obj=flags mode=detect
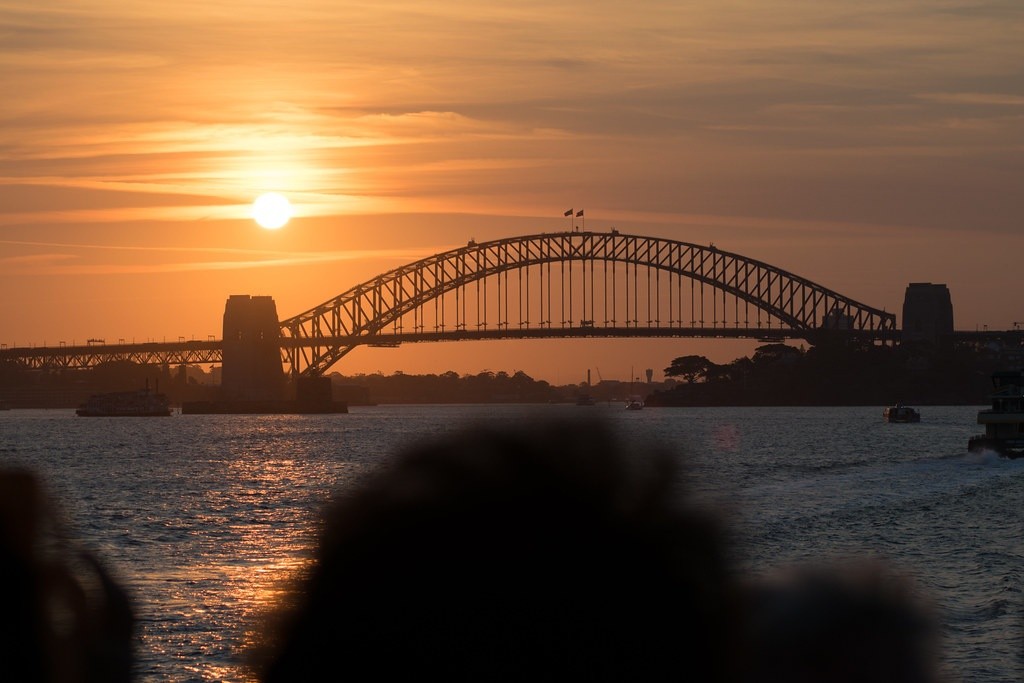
[576,210,583,217]
[564,208,573,216]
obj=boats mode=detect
[576,398,595,406]
[75,375,173,416]
[883,399,921,424]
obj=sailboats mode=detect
[625,366,642,410]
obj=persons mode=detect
[0,403,938,683]
